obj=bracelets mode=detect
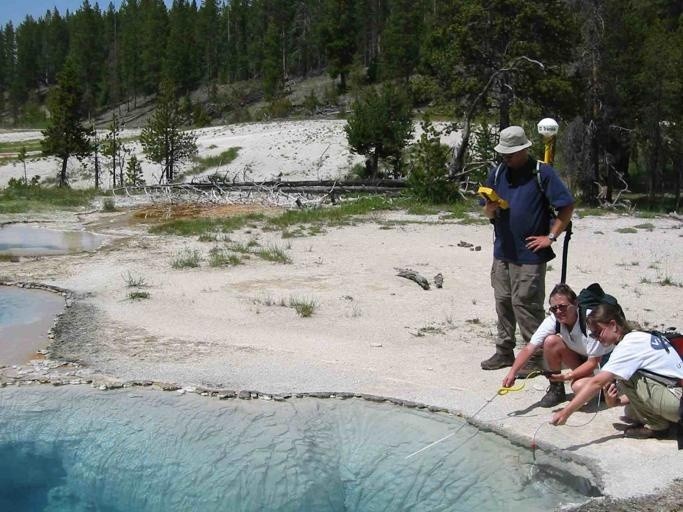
[567,372,572,380]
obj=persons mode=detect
[546,302,681,439]
[501,284,616,408]
[479,126,575,370]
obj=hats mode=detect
[493,125,532,154]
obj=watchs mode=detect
[548,232,555,241]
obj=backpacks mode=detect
[554,282,626,338]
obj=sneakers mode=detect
[513,348,544,379]
[480,349,515,370]
[624,424,670,440]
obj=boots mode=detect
[540,370,566,408]
